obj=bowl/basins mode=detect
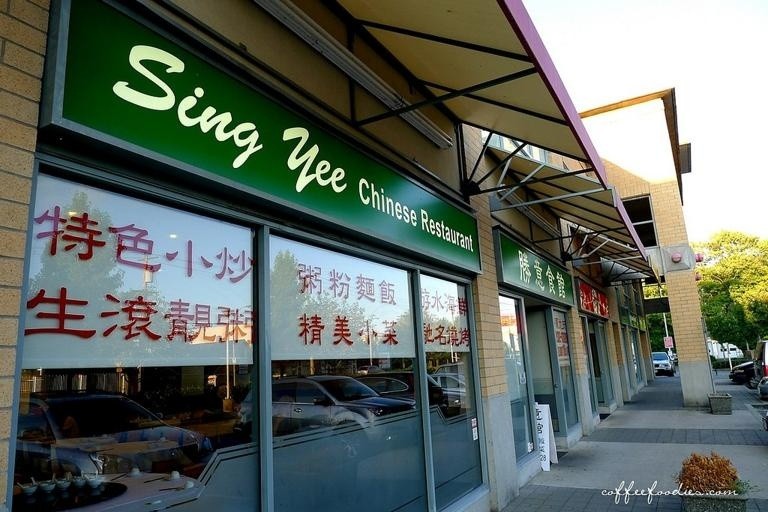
[21,476,102,496]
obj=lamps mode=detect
[497,183,566,241]
[258,0,455,151]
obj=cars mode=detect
[729,360,759,389]
[761,411,768,430]
[431,373,469,405]
[758,376,768,400]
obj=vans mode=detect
[750,340,767,388]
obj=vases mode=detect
[706,392,733,415]
[678,491,748,512]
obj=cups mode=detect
[171,470,180,478]
[160,437,165,441]
[130,467,140,475]
[184,481,193,490]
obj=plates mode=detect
[127,473,144,477]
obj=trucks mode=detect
[706,338,743,360]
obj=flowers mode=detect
[671,450,762,493]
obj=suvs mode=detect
[503,341,523,382]
[231,376,419,437]
[633,356,637,375]
[434,361,468,379]
[15,392,213,475]
[355,372,448,413]
[651,352,675,375]
